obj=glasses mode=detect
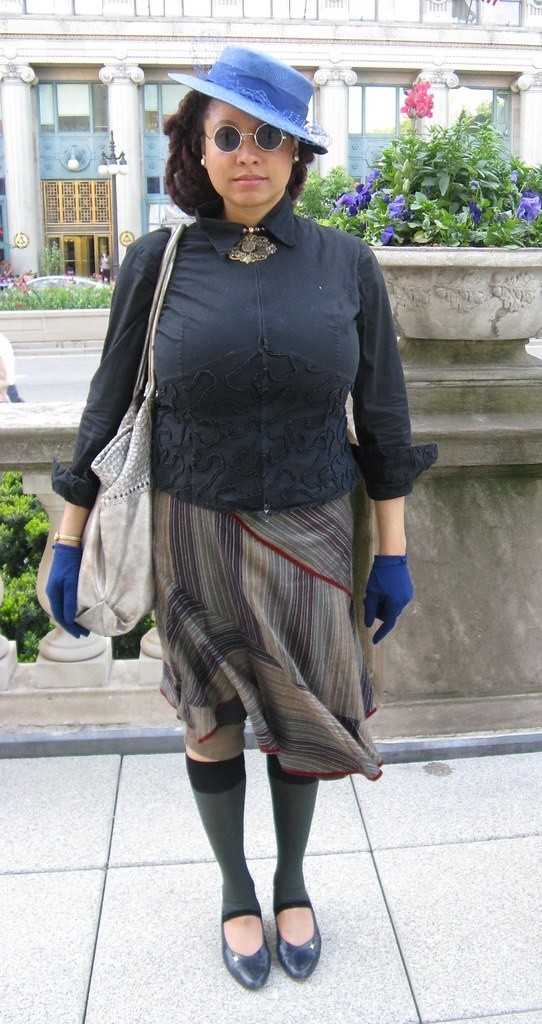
[204,122,288,153]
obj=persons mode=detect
[46,44,441,991]
[2,261,14,280]
[100,250,110,283]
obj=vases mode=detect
[368,249,542,342]
[0,309,113,347]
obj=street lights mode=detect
[97,130,128,281]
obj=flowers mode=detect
[0,278,111,311]
[309,80,542,249]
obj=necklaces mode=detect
[243,226,266,234]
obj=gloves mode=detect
[46,543,89,639]
[365,553,413,644]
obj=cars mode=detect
[23,277,110,294]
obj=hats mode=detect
[166,45,332,156]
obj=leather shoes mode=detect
[220,909,270,989]
[275,899,321,982]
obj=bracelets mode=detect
[53,531,82,541]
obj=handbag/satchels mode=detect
[74,383,158,637]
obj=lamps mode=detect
[66,145,78,171]
